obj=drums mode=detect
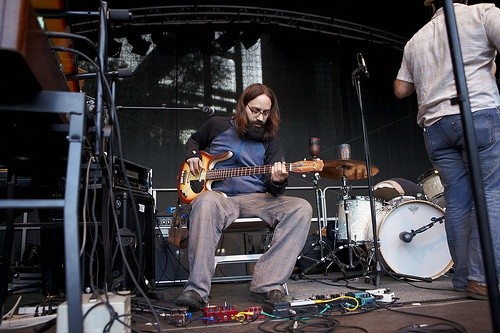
[417,169,447,209]
[369,197,455,281]
[335,195,385,244]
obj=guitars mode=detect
[176,150,324,204]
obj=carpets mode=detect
[282,270,468,306]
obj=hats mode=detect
[424,0,433,6]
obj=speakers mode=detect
[155,217,192,284]
[75,185,156,299]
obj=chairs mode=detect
[215,214,290,296]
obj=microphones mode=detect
[360,54,369,78]
[203,107,215,116]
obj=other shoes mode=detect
[174,290,206,310]
[465,281,488,300]
[250,289,293,306]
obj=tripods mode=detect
[293,172,376,280]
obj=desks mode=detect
[0,86,86,333]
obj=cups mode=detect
[338,144,351,160]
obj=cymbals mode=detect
[320,159,379,180]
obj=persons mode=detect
[395,0,500,301]
[173,83,313,310]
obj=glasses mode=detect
[248,104,272,117]
